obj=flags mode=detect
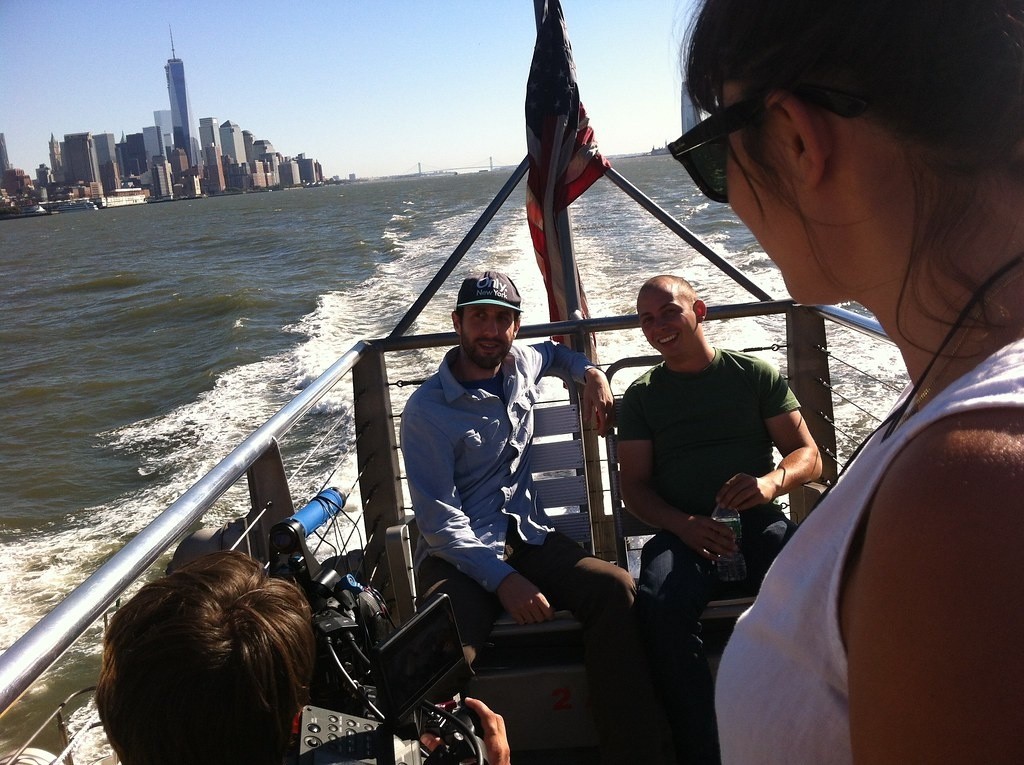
[522,0,609,354]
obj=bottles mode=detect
[712,501,748,584]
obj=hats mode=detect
[456,271,524,313]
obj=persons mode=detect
[399,271,666,763]
[96,552,511,765]
[671,0,1024,764]
[615,276,823,764]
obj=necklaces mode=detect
[887,246,1022,435]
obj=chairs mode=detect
[382,366,624,680]
[598,355,834,625]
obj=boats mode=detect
[50,198,98,213]
[99,186,148,208]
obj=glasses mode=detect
[667,84,871,204]
